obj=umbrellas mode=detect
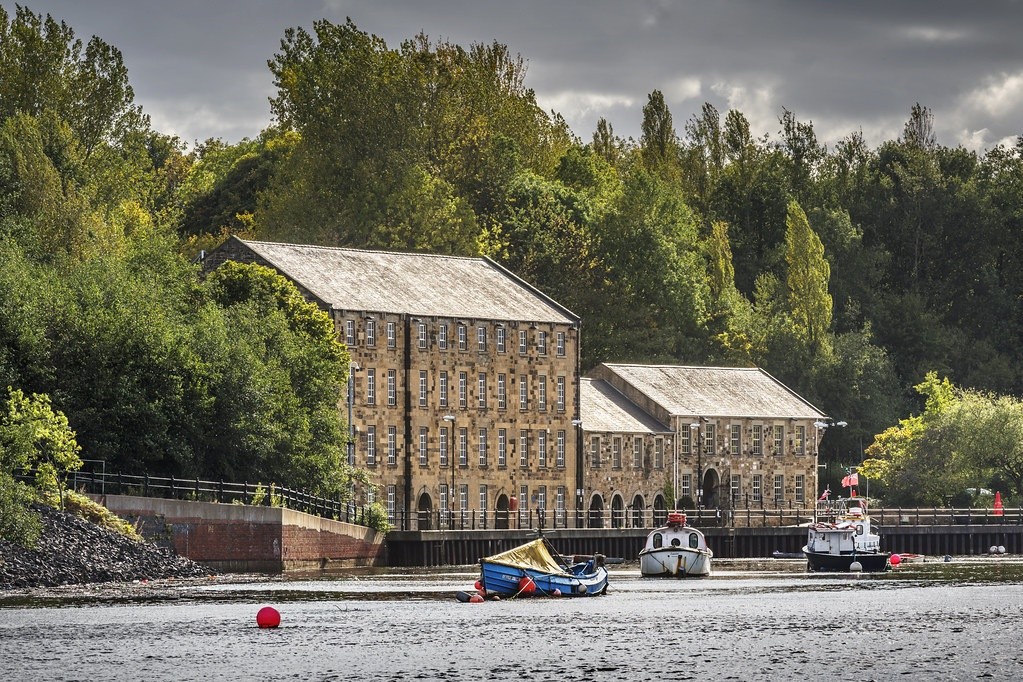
[994,491,1001,516]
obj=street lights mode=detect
[571,420,584,528]
[690,416,708,527]
[442,415,455,530]
[812,421,848,499]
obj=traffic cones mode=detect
[994,490,1003,514]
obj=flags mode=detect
[841,473,857,488]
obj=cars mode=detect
[950,488,993,508]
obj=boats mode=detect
[478,534,609,598]
[802,467,891,573]
[896,554,925,562]
[638,512,713,577]
